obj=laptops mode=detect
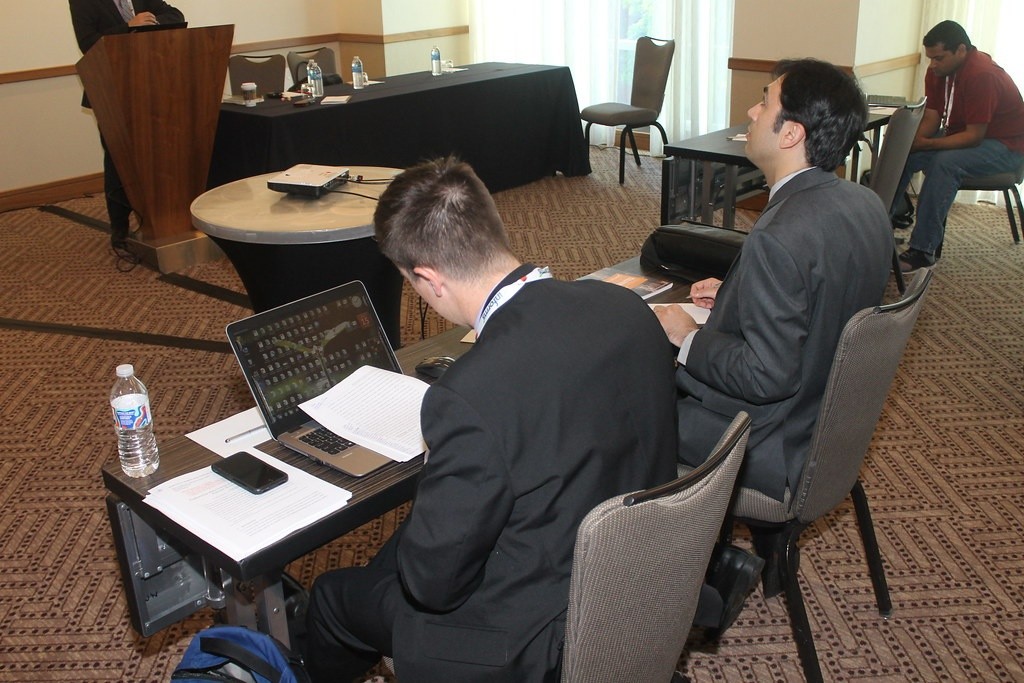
[224,279,402,475]
[128,22,189,33]
[851,65,907,106]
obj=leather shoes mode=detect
[751,520,800,597]
[707,542,764,640]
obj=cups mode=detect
[302,84,316,102]
[241,83,257,107]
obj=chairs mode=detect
[672,265,935,683]
[286,44,337,86]
[580,36,674,183]
[225,54,284,97]
[558,410,754,683]
[864,93,930,295]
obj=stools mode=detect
[933,171,1024,260]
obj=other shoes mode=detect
[111,220,129,248]
[890,247,938,274]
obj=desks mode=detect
[206,61,591,197]
[660,108,890,232]
[102,253,721,652]
[189,166,408,352]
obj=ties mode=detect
[119,0,134,24]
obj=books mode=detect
[732,133,747,142]
[223,95,264,106]
[647,303,711,324]
[281,92,309,98]
[320,96,351,105]
[570,267,674,301]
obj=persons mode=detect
[68,0,186,251]
[675,56,896,646]
[278,154,682,682]
[888,19,1024,274]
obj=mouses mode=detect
[415,355,455,378]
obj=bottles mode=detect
[352,56,364,89]
[109,364,160,479]
[431,45,441,76]
[306,59,323,97]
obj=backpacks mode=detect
[168,626,309,683]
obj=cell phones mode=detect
[212,451,288,494]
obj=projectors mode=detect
[267,164,349,198]
